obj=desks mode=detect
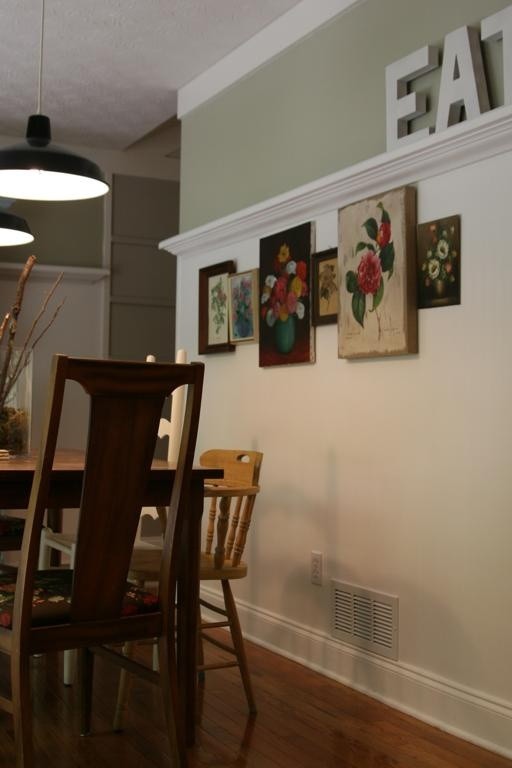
[1,437,226,766]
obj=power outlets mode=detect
[310,552,324,586]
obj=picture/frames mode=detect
[311,247,338,325]
[226,268,259,345]
[199,260,236,354]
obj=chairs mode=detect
[1,353,209,762]
[111,443,262,736]
[31,413,172,691]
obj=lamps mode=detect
[0,0,111,204]
[0,211,36,247]
